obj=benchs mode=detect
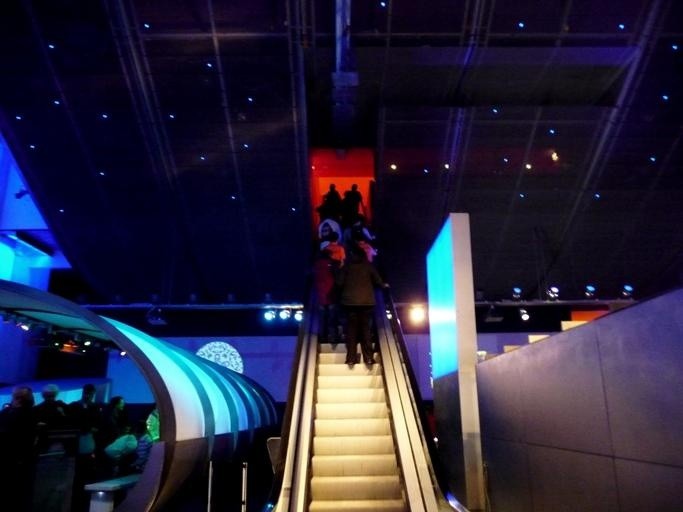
[84,474,144,512]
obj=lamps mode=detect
[511,284,634,300]
[3,313,34,333]
[147,308,168,327]
[518,306,531,322]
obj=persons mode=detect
[314,182,379,344]
[32,383,75,511]
[0,385,37,510]
[67,382,103,511]
[423,403,439,448]
[93,394,159,482]
[335,248,391,366]
